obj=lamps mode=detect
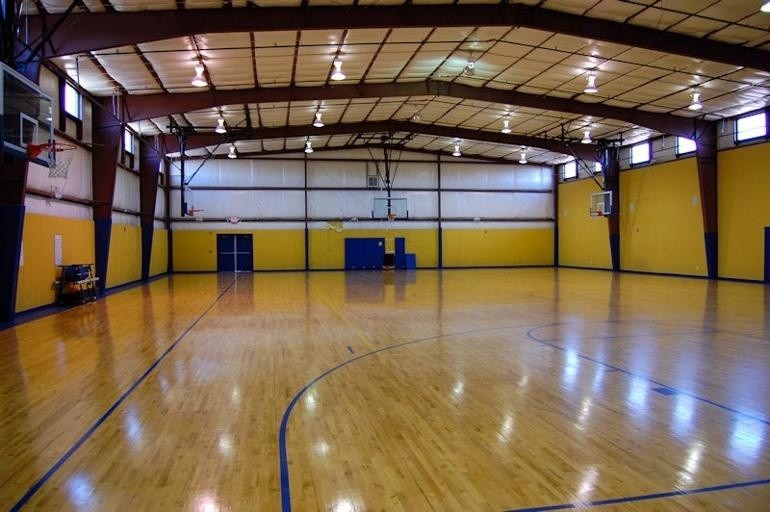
[451,115,529,164]
[579,125,592,144]
[688,87,704,110]
[412,109,420,123]
[190,63,237,159]
[583,72,597,94]
[464,61,475,77]
[305,58,347,154]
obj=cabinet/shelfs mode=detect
[53,263,99,304]
